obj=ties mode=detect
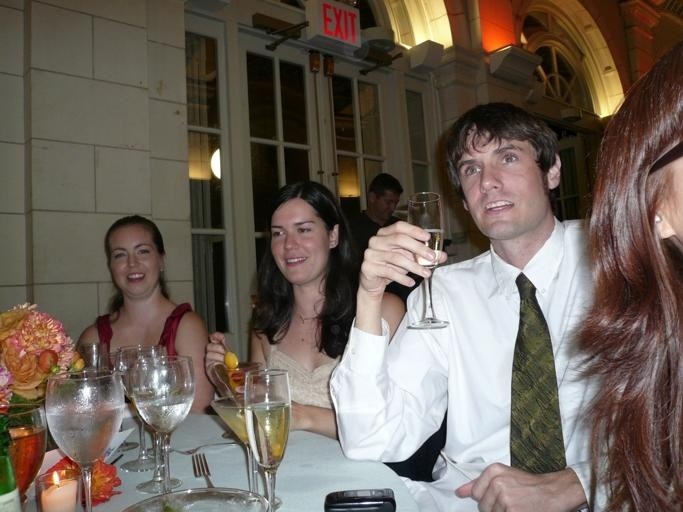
[508,271,568,476]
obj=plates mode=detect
[122,486,269,512]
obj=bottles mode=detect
[0,414,23,512]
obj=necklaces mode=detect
[298,316,315,324]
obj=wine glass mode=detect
[44,369,126,512]
[212,357,291,512]
[73,342,196,495]
[406,191,450,329]
[1,403,47,501]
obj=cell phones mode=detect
[324,488,397,512]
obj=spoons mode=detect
[219,339,239,369]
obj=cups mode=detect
[35,470,84,512]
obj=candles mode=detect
[40,468,78,511]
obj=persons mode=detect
[76,214,214,415]
[569,36,682,510]
[201,178,411,478]
[329,99,618,511]
[348,173,404,254]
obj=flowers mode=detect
[0,303,86,426]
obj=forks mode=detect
[191,452,214,488]
[174,442,241,455]
